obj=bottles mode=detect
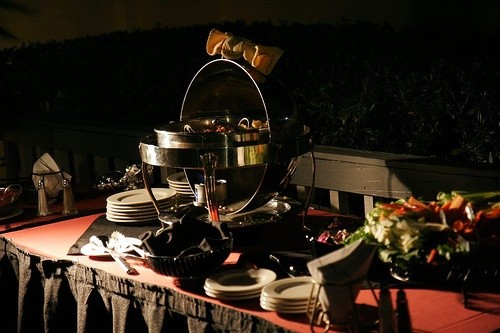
[63,178,77,214]
[396,290,412,333]
[37,180,52,216]
[379,288,395,333]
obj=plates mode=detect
[81,235,142,257]
[167,173,196,203]
[204,268,276,301]
[0,208,24,220]
[105,188,177,223]
[260,277,321,311]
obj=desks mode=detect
[0,211,500,333]
